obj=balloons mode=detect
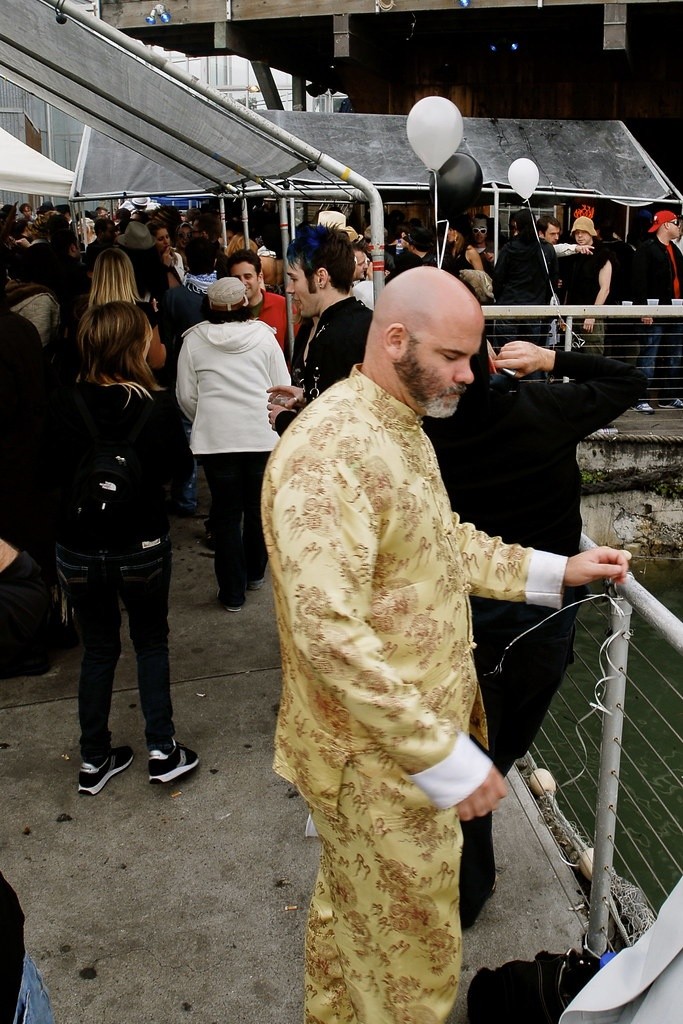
[507,158,539,199]
[406,96,464,173]
[429,152,483,221]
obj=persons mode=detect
[266,222,374,438]
[48,299,201,795]
[175,277,291,612]
[0,536,61,1024]
[262,267,632,1024]
[0,195,683,557]
[422,337,650,934]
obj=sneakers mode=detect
[148,738,199,786]
[83,745,135,799]
[631,402,655,415]
[657,396,683,408]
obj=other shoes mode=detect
[217,588,240,611]
[246,577,265,589]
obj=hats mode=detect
[116,221,156,249]
[403,227,435,249]
[308,211,358,243]
[132,196,150,207]
[570,216,598,237]
[648,211,677,234]
[207,277,248,311]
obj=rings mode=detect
[501,366,517,378]
[579,248,582,249]
[293,397,299,404]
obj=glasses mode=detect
[178,232,192,239]
[472,227,488,234]
[667,221,679,227]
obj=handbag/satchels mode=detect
[467,945,601,1024]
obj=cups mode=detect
[647,299,659,305]
[622,301,633,305]
[272,397,292,430]
[671,298,683,305]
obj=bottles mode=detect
[396,239,403,254]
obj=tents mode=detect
[0,0,683,362]
